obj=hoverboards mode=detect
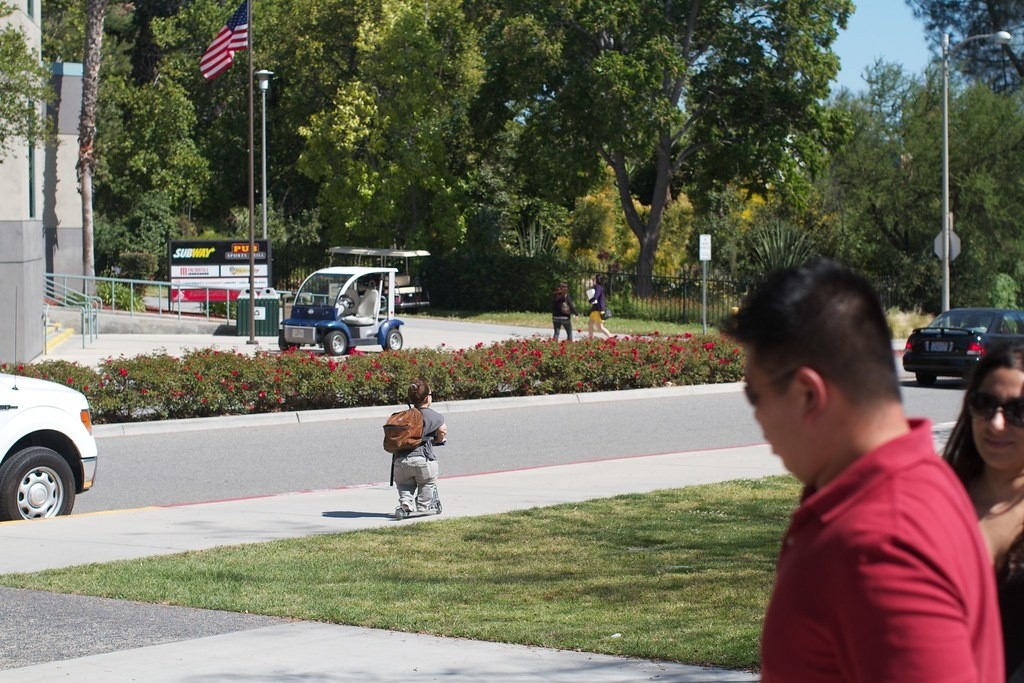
[393,439,446,521]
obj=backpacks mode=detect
[382,408,431,453]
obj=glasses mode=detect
[744,372,794,406]
[967,392,1024,428]
[428,394,434,396]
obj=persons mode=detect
[552,283,578,341]
[588,275,613,338]
[941,350,1024,683]
[394,382,447,512]
[719,266,1005,683]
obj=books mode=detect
[587,289,597,304]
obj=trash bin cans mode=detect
[236,287,280,337]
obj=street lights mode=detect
[254,68,274,241]
[941,29,1013,325]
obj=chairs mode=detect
[343,280,379,325]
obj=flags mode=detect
[200,0,249,80]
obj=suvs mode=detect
[0,372,100,523]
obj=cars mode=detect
[902,306,1024,389]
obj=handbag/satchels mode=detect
[562,293,572,315]
[602,307,612,320]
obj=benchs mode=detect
[384,275,423,294]
